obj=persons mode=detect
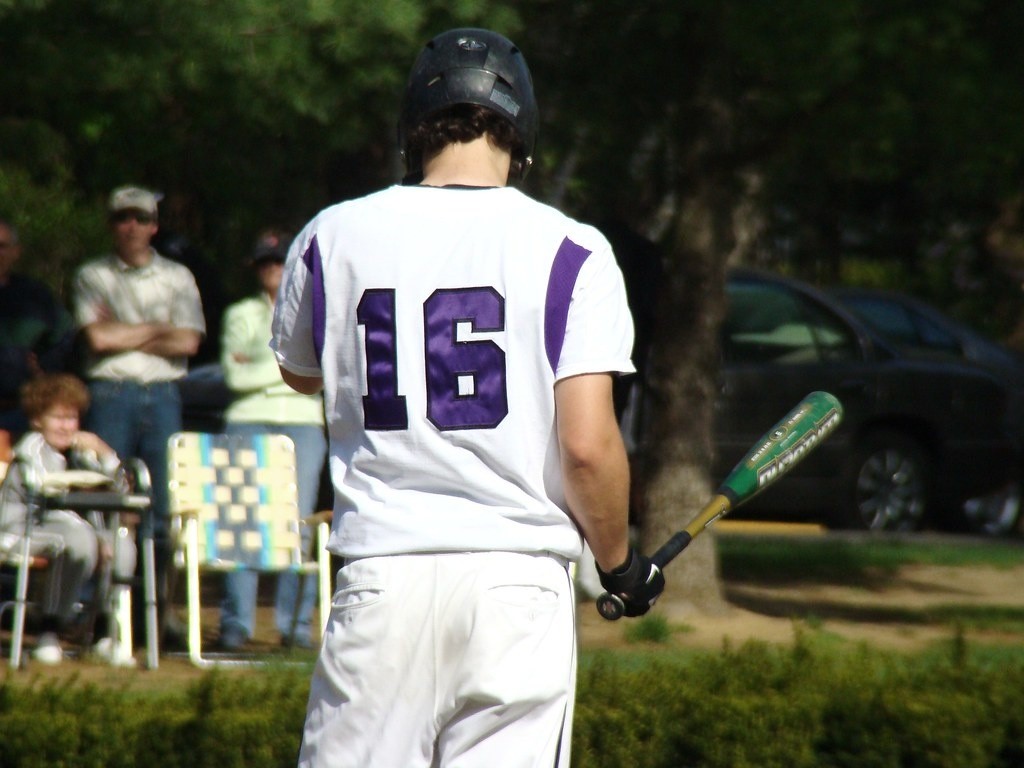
[270,26,664,768]
[218,230,328,653]
[70,186,206,648]
[0,372,141,667]
[0,222,75,477]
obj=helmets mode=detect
[398,28,538,181]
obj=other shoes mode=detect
[30,626,64,666]
[219,624,250,650]
[88,639,137,668]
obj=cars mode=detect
[724,262,1024,537]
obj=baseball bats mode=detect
[596,391,843,622]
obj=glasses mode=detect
[258,255,285,265]
[111,210,154,223]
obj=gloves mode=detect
[596,544,665,617]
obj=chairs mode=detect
[0,430,333,672]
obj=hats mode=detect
[108,184,162,210]
[252,230,293,257]
